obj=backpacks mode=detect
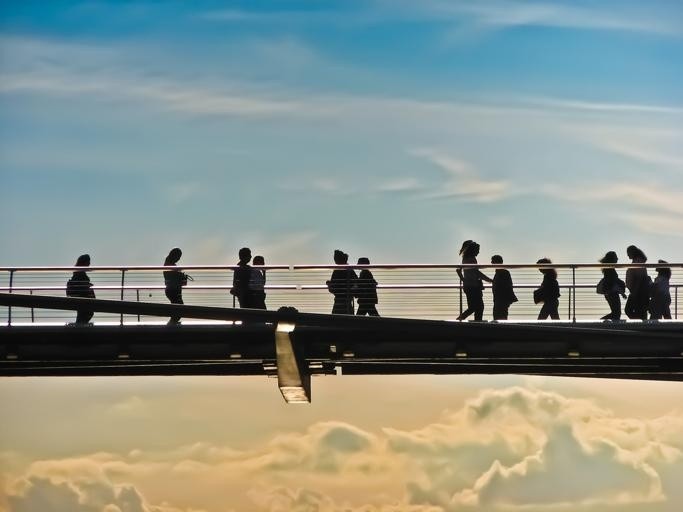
[455,266,466,282]
[348,270,358,298]
[66,275,80,297]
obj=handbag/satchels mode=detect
[88,284,96,298]
[648,276,654,290]
[178,272,193,286]
[534,288,544,304]
[625,294,646,319]
[597,278,625,294]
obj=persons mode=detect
[325,249,358,315]
[648,260,671,319]
[248,255,267,325]
[626,245,648,320]
[350,257,379,316]
[490,255,518,320]
[162,247,183,321]
[229,247,252,326]
[599,251,626,320]
[65,254,96,322]
[533,259,560,320]
[456,240,493,321]
